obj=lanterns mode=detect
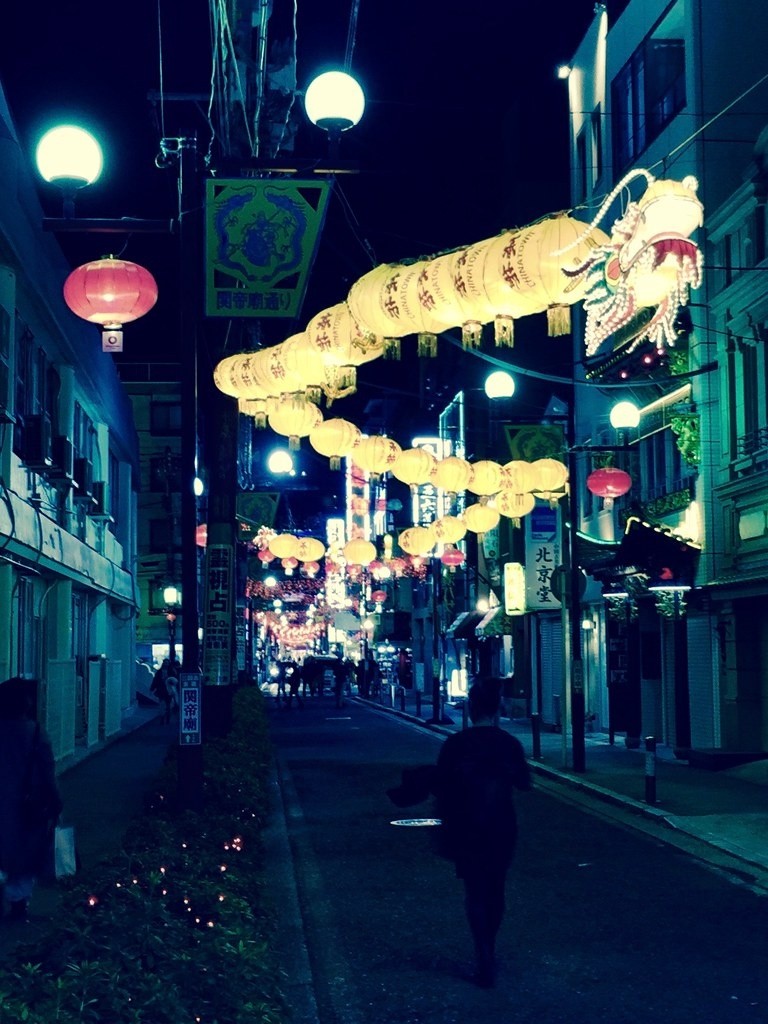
[214,210,632,613]
[196,523,207,554]
[63,254,158,352]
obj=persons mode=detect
[149,656,386,728]
[426,679,533,989]
[0,677,66,923]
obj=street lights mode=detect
[484,371,640,770]
[31,69,368,780]
